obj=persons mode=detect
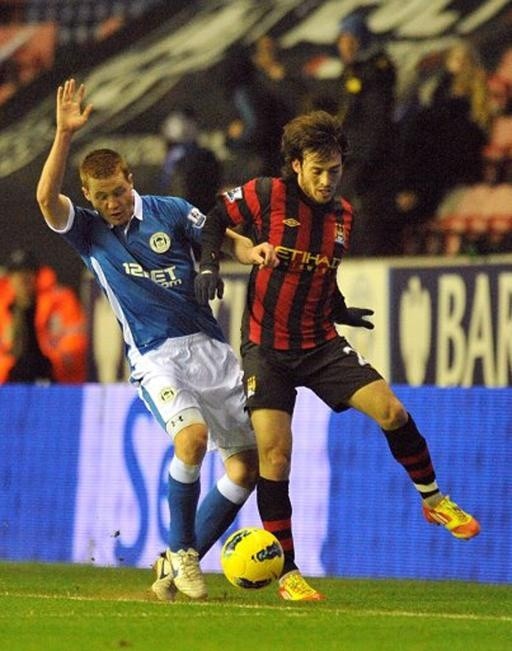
[0,245,96,389]
[195,108,481,603]
[151,16,512,258]
[34,76,260,601]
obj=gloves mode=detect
[194,265,224,304]
[335,303,374,329]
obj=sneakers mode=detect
[152,557,177,602]
[278,569,327,602]
[423,494,480,539]
[167,547,208,598]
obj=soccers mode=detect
[224,528,285,590]
